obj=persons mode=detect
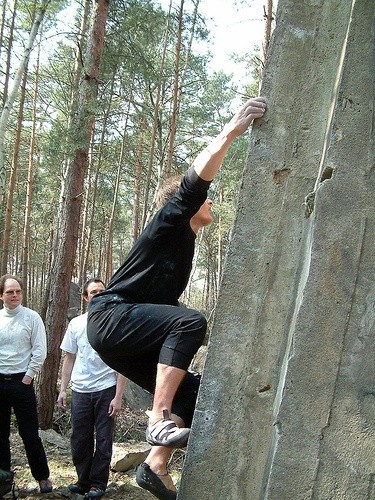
[86,96,267,500]
[57,278,127,500]
[0,274,53,497]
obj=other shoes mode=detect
[136,463,177,500]
[86,487,105,496]
[146,410,190,448]
[39,480,53,493]
[68,484,89,497]
